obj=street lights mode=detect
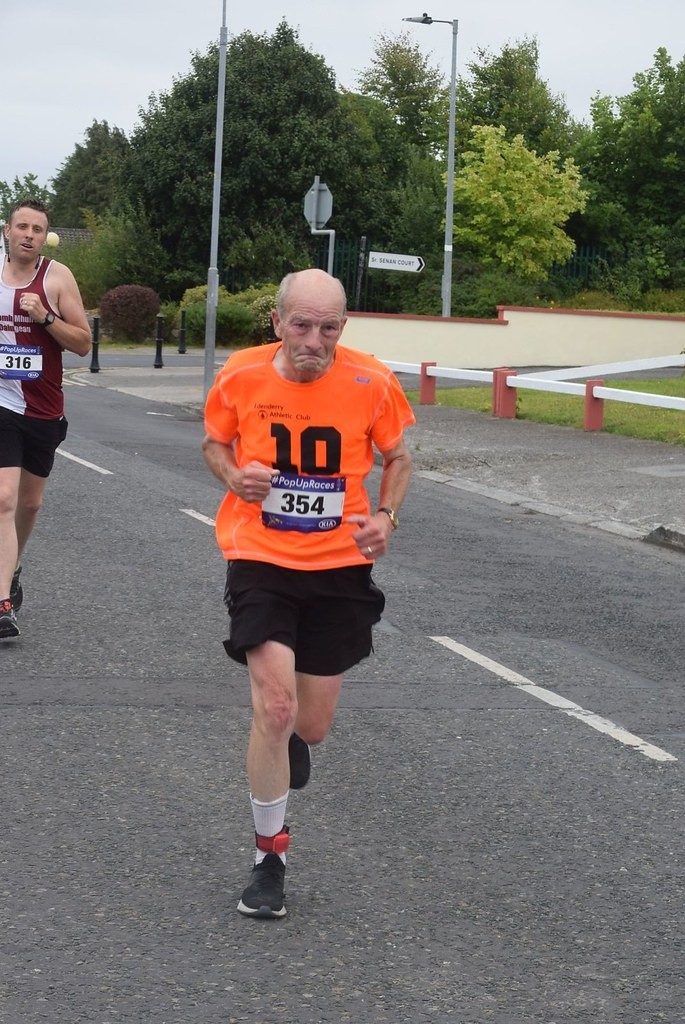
[46,232,59,259]
[404,16,458,318]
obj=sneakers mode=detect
[235,825,290,917]
[0,565,23,638]
[288,732,311,790]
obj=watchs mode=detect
[41,312,55,327]
[378,508,400,529]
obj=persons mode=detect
[0,201,92,639]
[203,269,416,919]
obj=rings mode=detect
[367,546,373,553]
